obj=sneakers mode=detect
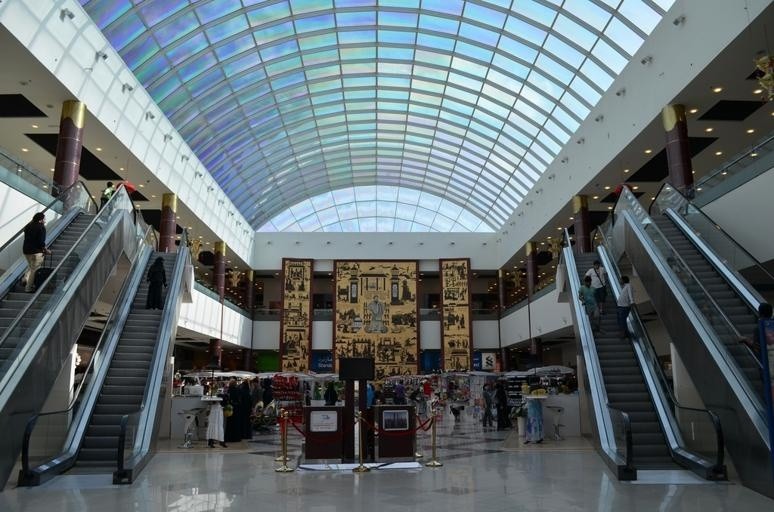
[19,281,27,287]
[25,288,35,292]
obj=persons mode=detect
[96,182,121,217]
[523,400,543,444]
[225,376,464,442]
[146,256,167,310]
[206,402,228,448]
[22,212,50,292]
[578,277,600,338]
[616,275,633,338]
[494,383,513,432]
[483,383,493,427]
[584,260,608,312]
[737,303,774,401]
[368,295,385,331]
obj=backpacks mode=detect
[101,187,113,200]
[150,265,163,282]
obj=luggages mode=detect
[34,253,56,294]
